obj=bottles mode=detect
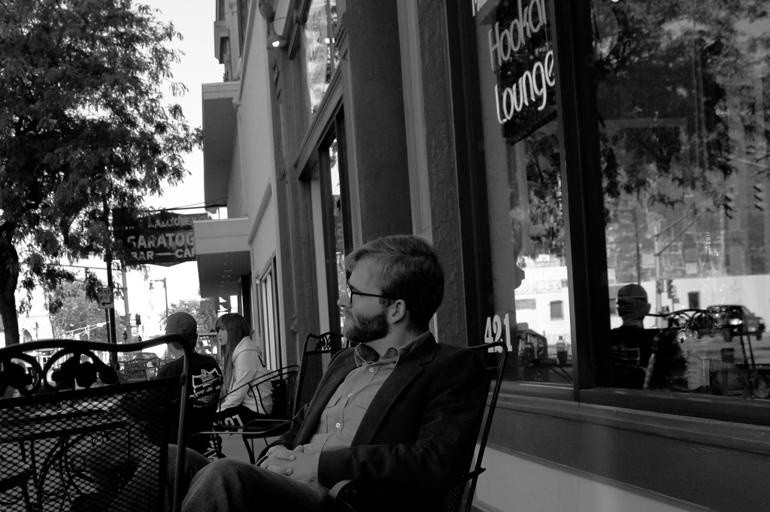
[556,336,568,364]
[711,346,751,397]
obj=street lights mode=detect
[138,336,142,353]
[122,331,128,361]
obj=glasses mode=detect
[344,279,398,307]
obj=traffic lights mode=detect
[656,279,664,294]
[666,279,676,297]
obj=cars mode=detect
[692,305,765,342]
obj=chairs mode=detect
[0,329,509,510]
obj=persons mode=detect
[117,312,223,452]
[610,284,690,393]
[107,234,492,512]
[209,313,274,428]
